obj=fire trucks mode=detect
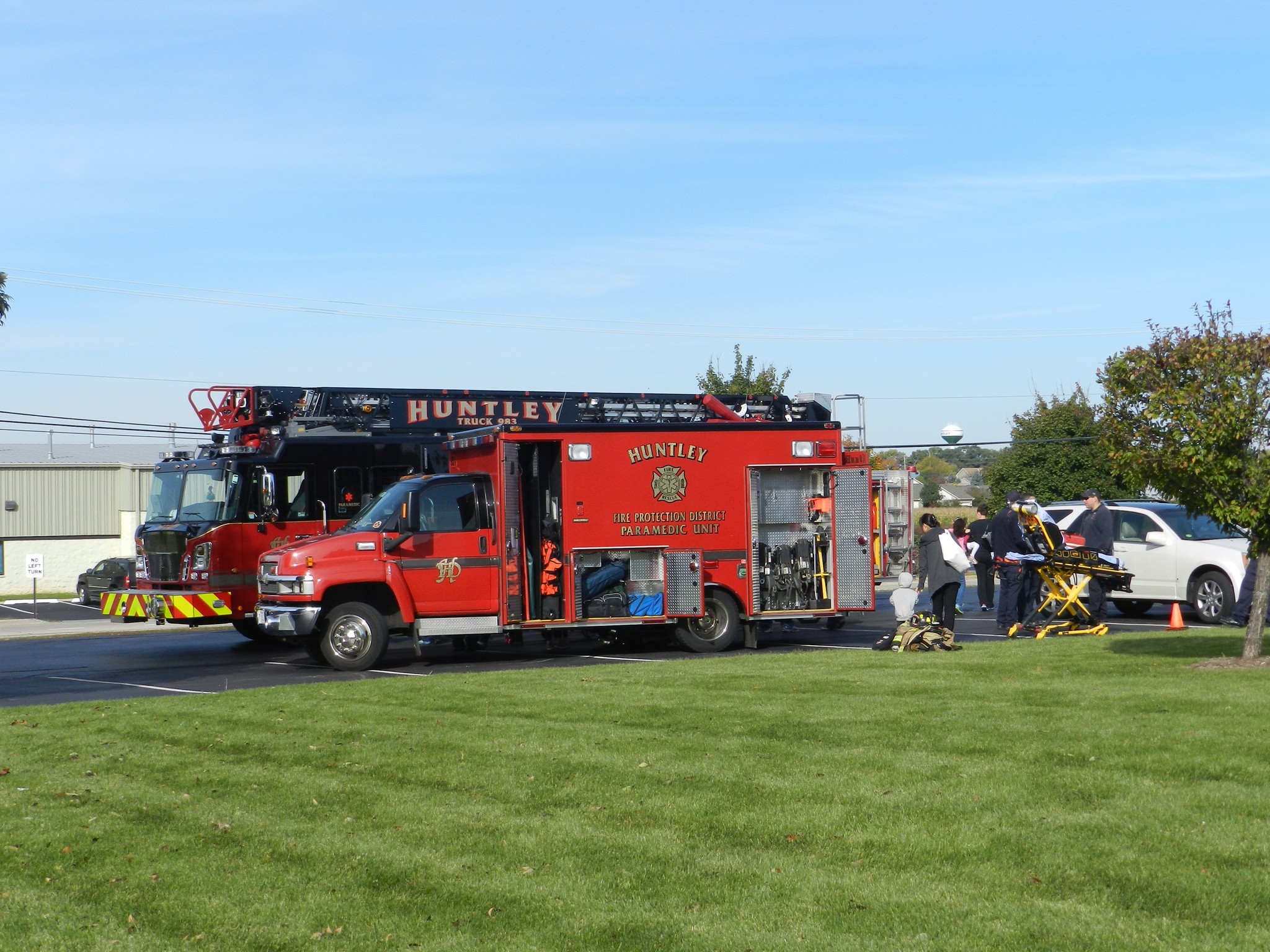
[254,394,920,677]
[98,380,866,646]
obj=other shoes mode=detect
[997,625,1004,629]
[1005,625,1010,630]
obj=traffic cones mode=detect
[1165,603,1189,630]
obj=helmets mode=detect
[910,610,940,628]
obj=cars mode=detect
[76,556,138,605]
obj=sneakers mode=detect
[968,556,978,564]
[955,604,964,615]
[981,604,989,611]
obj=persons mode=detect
[947,489,1065,630]
[1079,488,1114,626]
[889,572,919,628]
[1217,508,1270,628]
[915,513,961,632]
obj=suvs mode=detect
[1042,499,1251,625]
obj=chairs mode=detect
[1122,521,1143,541]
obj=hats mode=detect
[1006,490,1025,501]
[1079,488,1101,500]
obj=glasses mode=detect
[1082,497,1093,500]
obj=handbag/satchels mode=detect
[581,558,629,600]
[981,525,992,553]
[627,592,663,616]
[582,589,628,618]
[939,528,971,573]
